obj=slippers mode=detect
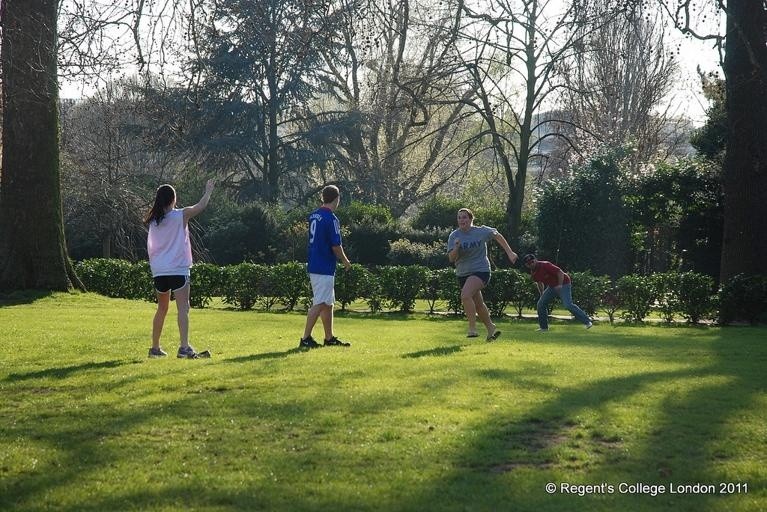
[466,331,482,338]
[484,331,502,343]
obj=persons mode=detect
[446,208,520,341]
[143,175,221,360]
[523,251,595,333]
[298,182,354,350]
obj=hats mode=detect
[522,253,536,263]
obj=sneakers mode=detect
[299,335,323,348]
[177,345,197,358]
[323,336,351,347]
[585,317,593,329]
[536,326,550,333]
[147,348,168,358]
